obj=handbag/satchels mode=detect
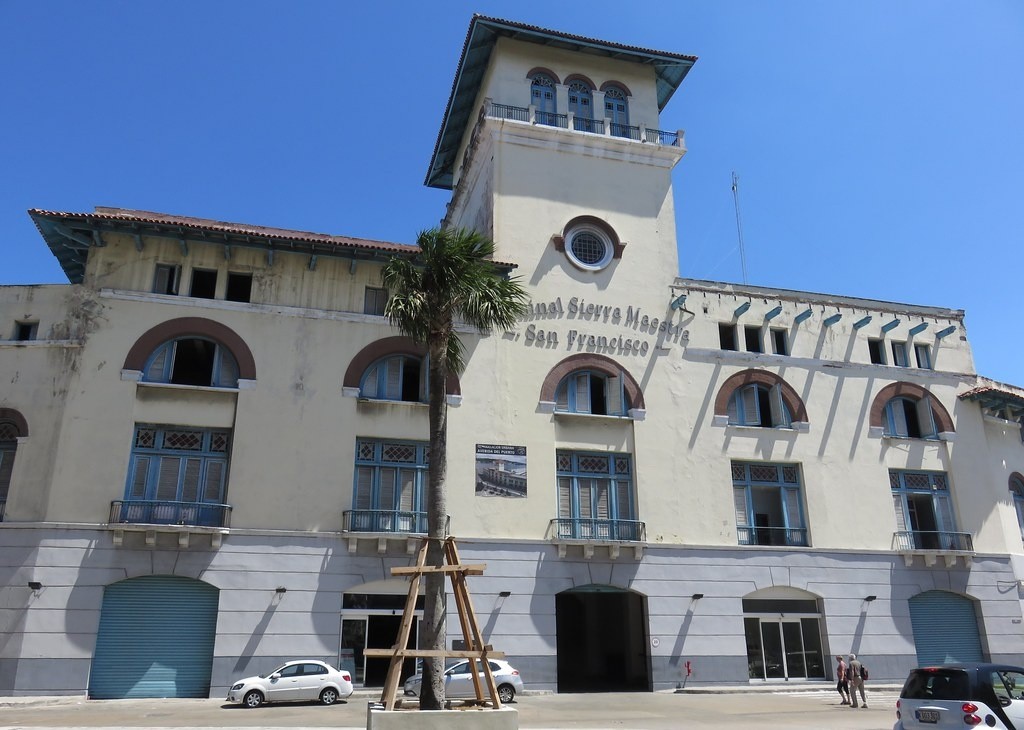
[861,664,869,681]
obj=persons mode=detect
[848,654,868,708]
[836,655,851,705]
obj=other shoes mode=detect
[849,703,858,707]
[861,704,868,708]
[841,701,851,705]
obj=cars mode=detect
[404,658,524,705]
[225,660,354,708]
[893,662,1024,730]
